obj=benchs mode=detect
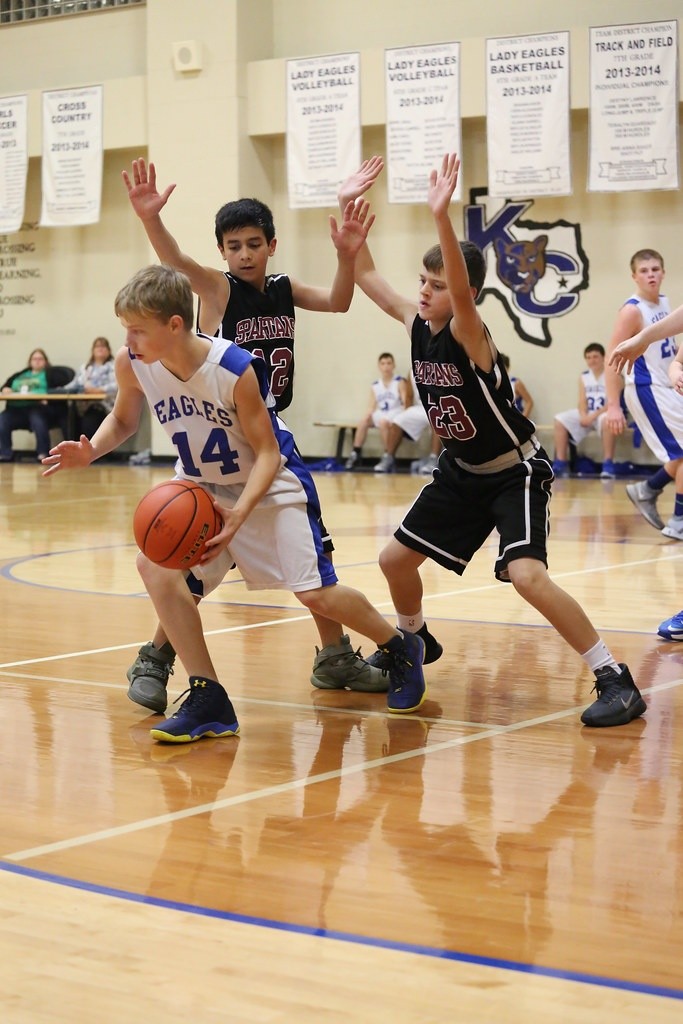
[533,425,640,476]
[312,422,361,467]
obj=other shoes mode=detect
[36,451,49,463]
[0,454,15,463]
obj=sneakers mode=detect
[552,460,570,479]
[373,454,396,472]
[364,621,444,670]
[579,719,647,762]
[598,459,616,478]
[382,714,427,775]
[657,608,683,641]
[126,642,177,712]
[149,736,240,787]
[627,479,664,530]
[343,449,361,468]
[310,692,387,732]
[151,675,238,742]
[311,636,391,692]
[422,454,438,474]
[375,625,427,714]
[661,516,683,541]
[581,662,646,727]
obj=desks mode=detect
[0,391,106,443]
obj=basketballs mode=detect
[131,478,224,571]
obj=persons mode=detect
[56,337,119,464]
[0,349,68,462]
[551,342,629,478]
[373,366,443,473]
[344,352,408,474]
[336,152,646,728]
[499,353,534,417]
[121,157,390,713]
[41,265,427,743]
[608,304,683,396]
[603,248,683,531]
